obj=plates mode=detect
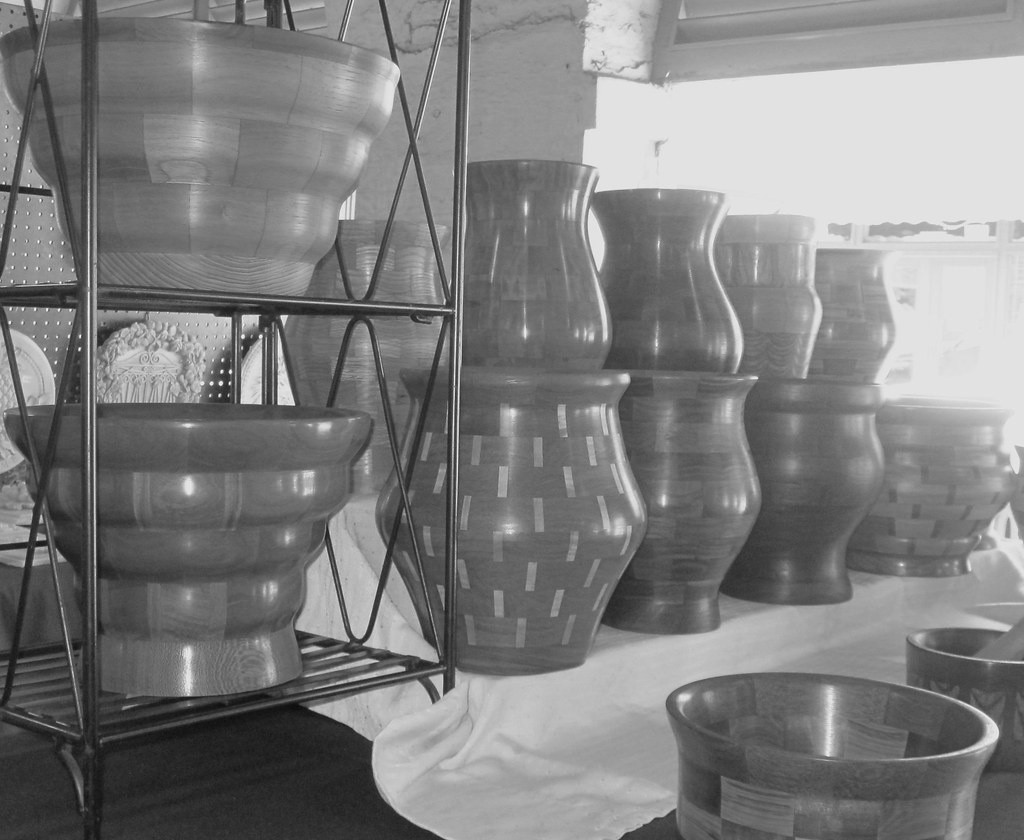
[241,330,295,407]
[0,328,55,474]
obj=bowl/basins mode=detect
[665,672,999,840]
[906,626,1024,775]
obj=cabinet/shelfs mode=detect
[0,0,474,840]
